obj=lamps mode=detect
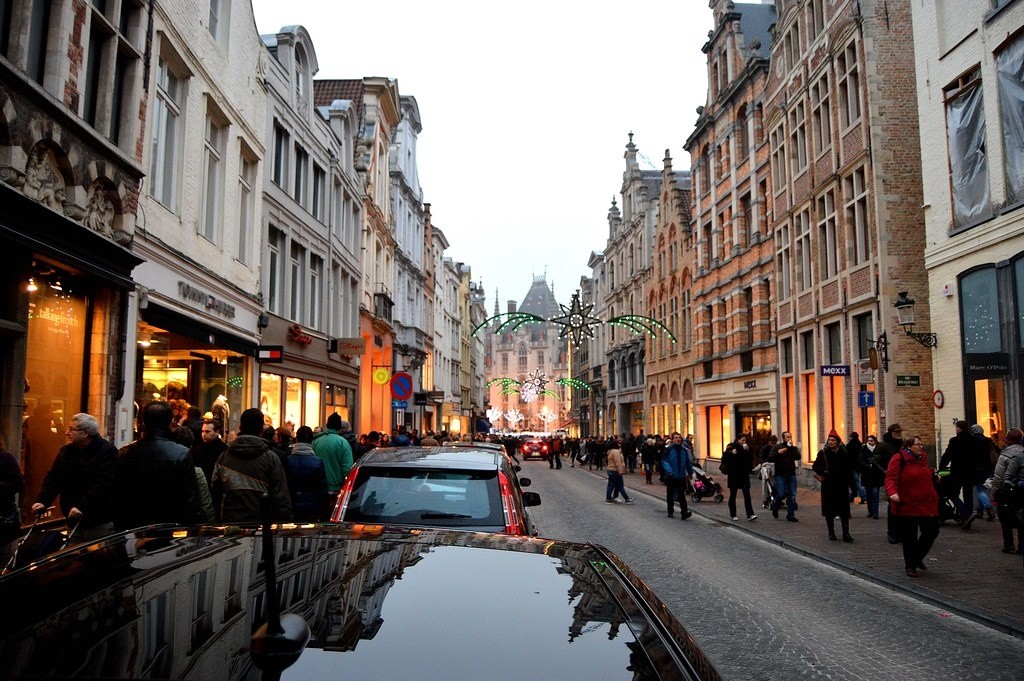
[894,291,937,348]
[138,287,148,310]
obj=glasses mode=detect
[912,442,925,446]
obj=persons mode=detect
[719,431,802,524]
[987,427,1024,554]
[660,431,692,520]
[604,440,636,505]
[542,429,695,485]
[884,436,938,577]
[0,401,359,551]
[812,424,904,542]
[938,420,1001,530]
[358,428,523,465]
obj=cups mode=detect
[795,460,800,467]
[743,443,748,449]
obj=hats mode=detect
[888,423,903,432]
[971,424,984,435]
[1002,427,1023,444]
[828,430,841,443]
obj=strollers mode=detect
[760,462,799,511]
[0,506,84,575]
[931,466,963,522]
[685,462,724,503]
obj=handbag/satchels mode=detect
[813,449,829,482]
[719,443,734,475]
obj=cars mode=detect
[440,440,532,490]
[0,519,721,681]
[521,438,549,461]
[329,445,541,537]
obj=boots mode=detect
[974,507,984,519]
[986,507,995,521]
[645,473,652,485]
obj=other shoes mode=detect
[888,533,898,545]
[681,512,692,520]
[1001,546,1016,553]
[842,532,855,542]
[786,515,799,522]
[614,498,623,503]
[730,516,739,521]
[772,507,779,519]
[604,499,618,504]
[828,531,836,541]
[748,515,757,521]
[867,512,878,520]
[625,498,636,504]
[859,498,867,504]
[916,561,927,570]
[668,511,673,517]
[906,566,917,578]
[961,516,974,530]
